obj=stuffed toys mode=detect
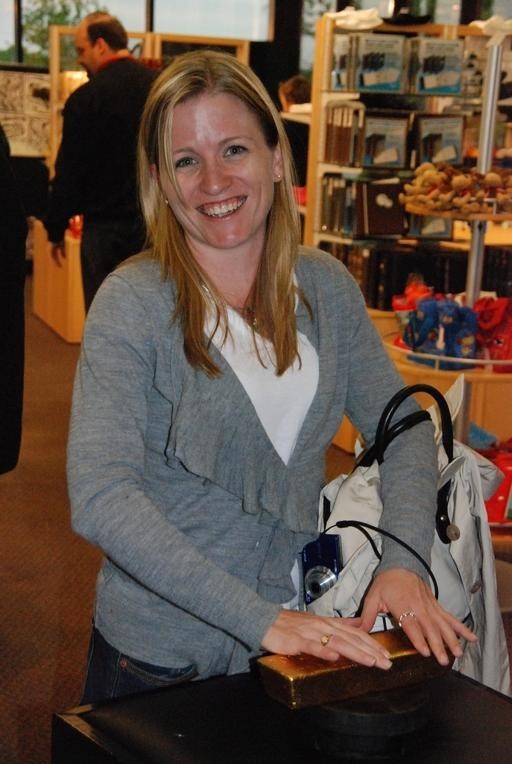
[401,163,512,214]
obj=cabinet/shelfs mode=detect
[51,651,512,762]
[30,217,86,346]
[302,15,512,454]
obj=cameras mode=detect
[302,533,343,604]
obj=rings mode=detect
[321,634,333,646]
[399,608,414,627]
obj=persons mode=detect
[64,48,477,706]
[44,10,163,320]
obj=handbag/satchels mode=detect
[402,297,478,370]
[322,382,471,633]
[473,296,512,375]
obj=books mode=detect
[412,37,463,95]
[449,251,512,296]
[321,177,407,241]
[325,107,408,172]
[410,111,465,169]
[319,241,448,311]
[410,214,454,241]
[333,33,407,95]
